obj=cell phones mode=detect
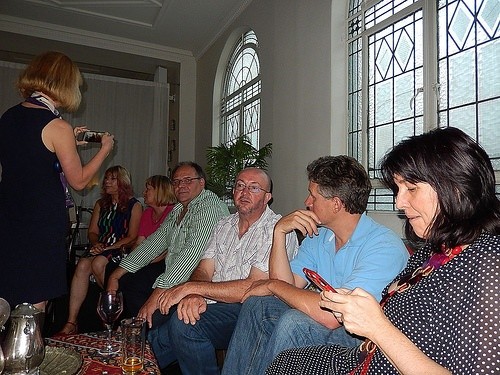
[303,268,338,293]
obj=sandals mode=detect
[54,323,78,337]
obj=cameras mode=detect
[77,130,110,143]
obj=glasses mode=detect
[235,181,268,193]
[171,178,202,185]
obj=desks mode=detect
[41,331,162,375]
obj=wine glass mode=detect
[97,292,123,353]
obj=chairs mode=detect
[68,205,93,274]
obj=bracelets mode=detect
[104,246,107,251]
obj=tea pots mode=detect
[2,303,45,375]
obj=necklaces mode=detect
[155,214,162,222]
[442,246,462,264]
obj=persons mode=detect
[104,175,175,291]
[108,161,231,338]
[56,165,143,335]
[221,156,410,375]
[0,51,114,338]
[147,168,300,375]
[264,126,500,375]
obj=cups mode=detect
[121,319,143,373]
